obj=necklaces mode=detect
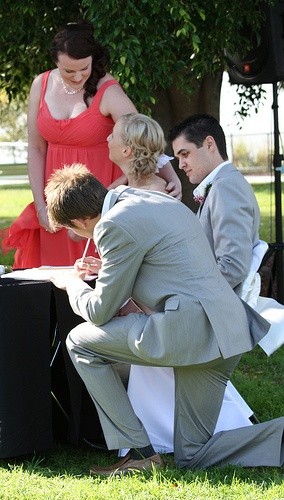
[60,78,85,96]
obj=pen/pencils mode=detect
[77,238,90,273]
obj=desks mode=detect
[0,267,100,455]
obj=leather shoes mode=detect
[90,450,164,475]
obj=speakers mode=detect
[224,0,284,88]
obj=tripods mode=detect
[254,81,284,305]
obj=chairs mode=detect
[232,244,282,303]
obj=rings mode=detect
[86,263,89,272]
[177,196,182,201]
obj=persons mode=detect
[0,46,182,268]
[105,111,169,196]
[78,112,259,453]
[42,160,284,480]
